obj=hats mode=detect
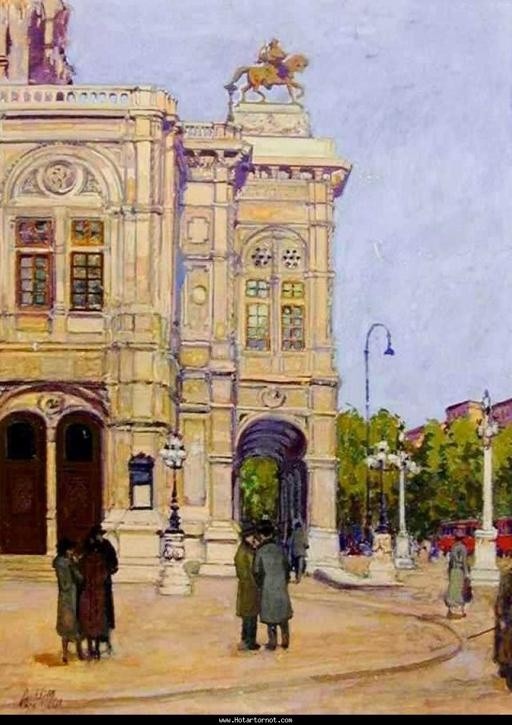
[256,519,276,533]
[55,534,82,551]
[82,524,108,541]
[238,521,257,537]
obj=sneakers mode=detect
[238,642,289,651]
[61,644,111,663]
[447,611,467,618]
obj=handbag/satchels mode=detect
[462,576,472,603]
